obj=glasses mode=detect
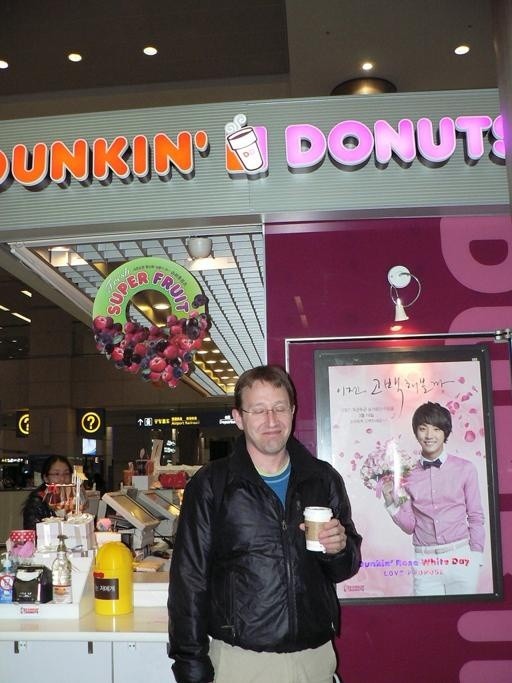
[48,471,70,476]
[237,402,292,418]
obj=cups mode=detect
[227,127,265,175]
[303,506,334,552]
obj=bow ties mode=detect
[423,458,442,469]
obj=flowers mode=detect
[360,438,411,507]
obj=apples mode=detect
[158,470,188,489]
[93,316,208,389]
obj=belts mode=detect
[415,539,469,554]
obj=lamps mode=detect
[388,266,421,322]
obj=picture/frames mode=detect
[315,344,504,606]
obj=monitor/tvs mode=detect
[101,491,160,531]
[139,490,181,521]
[81,438,97,457]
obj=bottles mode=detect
[93,534,134,617]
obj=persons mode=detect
[19,454,89,536]
[165,365,363,681]
[370,400,486,597]
